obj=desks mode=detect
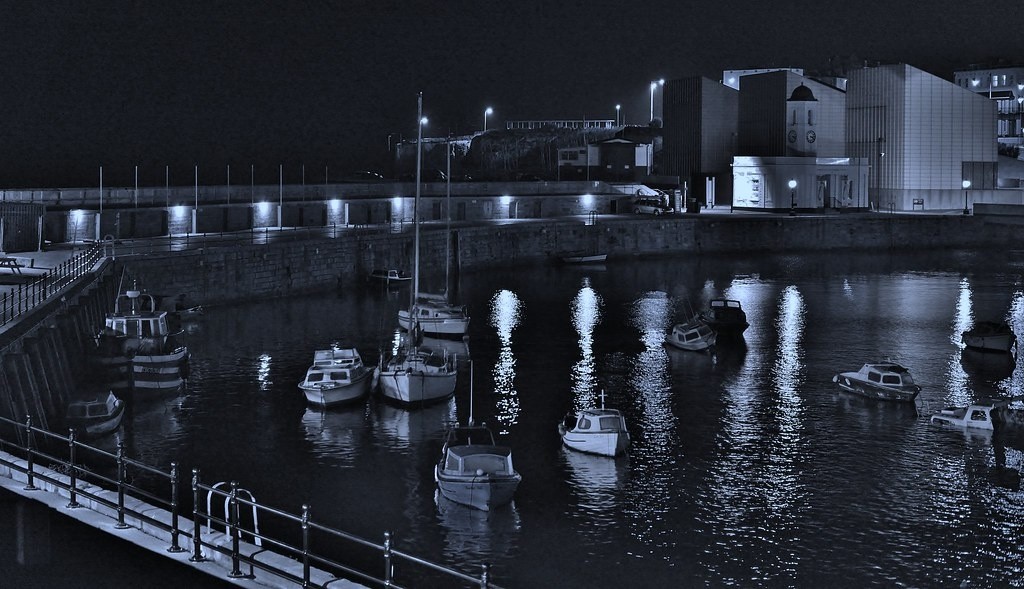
[0,257,22,275]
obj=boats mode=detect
[699,299,750,337]
[303,407,366,464]
[930,406,1001,433]
[433,490,522,558]
[663,320,717,351]
[370,268,412,288]
[961,323,1017,352]
[83,269,191,398]
[557,387,630,458]
[167,295,204,320]
[563,252,608,262]
[64,388,124,439]
[434,360,523,512]
[561,445,629,489]
[833,362,920,403]
[296,348,377,407]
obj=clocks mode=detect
[806,130,816,143]
[788,130,797,143]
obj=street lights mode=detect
[650,78,664,123]
[788,178,798,215]
[615,104,621,127]
[484,108,492,131]
[962,179,971,214]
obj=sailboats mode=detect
[396,127,469,339]
[377,397,460,543]
[376,90,459,407]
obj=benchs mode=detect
[0,264,25,268]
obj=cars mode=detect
[632,200,675,216]
